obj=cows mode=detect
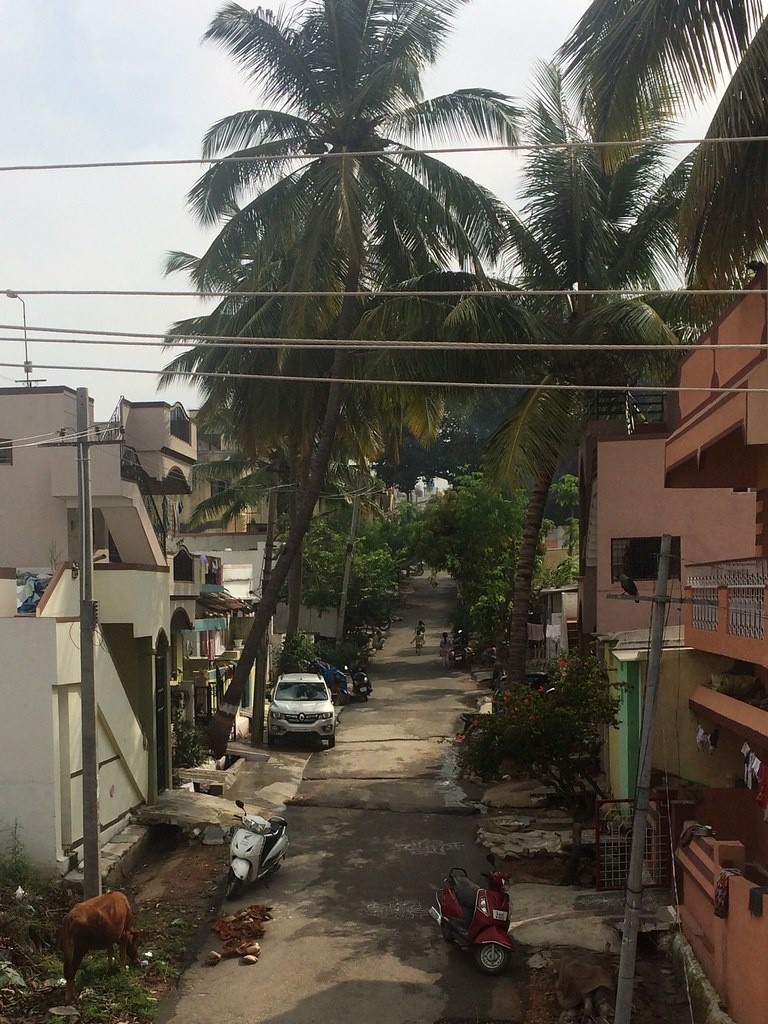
[59,891,144,1003]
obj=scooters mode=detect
[226,799,289,901]
[345,659,373,702]
[447,628,466,669]
[427,852,517,976]
[409,560,426,576]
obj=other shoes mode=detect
[416,651,417,654]
[418,653,420,656]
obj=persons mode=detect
[480,644,497,668]
[440,632,452,670]
[410,620,426,655]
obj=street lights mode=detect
[6,288,29,388]
[605,530,720,1023]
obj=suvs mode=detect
[267,670,338,747]
